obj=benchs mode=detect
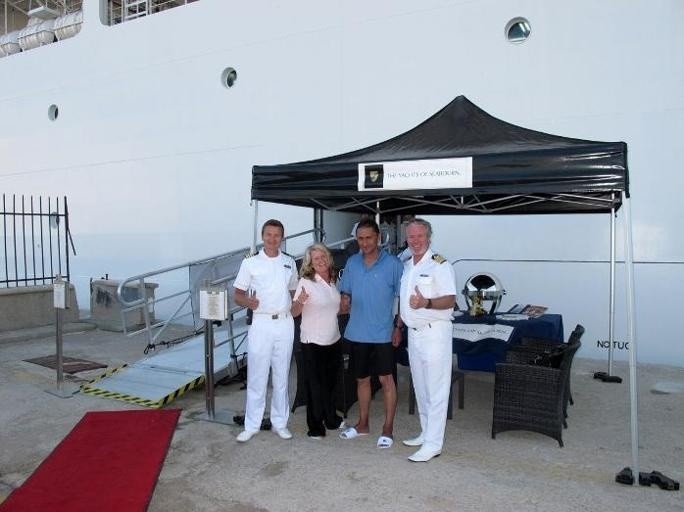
[286,312,395,420]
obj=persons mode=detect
[290,241,349,441]
[395,217,458,464]
[334,217,404,448]
[231,220,299,442]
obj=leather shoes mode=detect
[403,436,424,446]
[408,447,441,461]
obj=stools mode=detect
[405,365,466,422]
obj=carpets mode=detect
[0,404,183,512]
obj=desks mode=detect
[444,307,565,373]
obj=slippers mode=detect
[339,427,369,439]
[377,437,393,448]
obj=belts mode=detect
[254,312,292,319]
[412,320,446,331]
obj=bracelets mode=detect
[425,299,432,310]
[394,324,401,329]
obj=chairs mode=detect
[517,322,584,420]
[487,338,584,452]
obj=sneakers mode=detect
[272,426,292,439]
[237,430,255,441]
